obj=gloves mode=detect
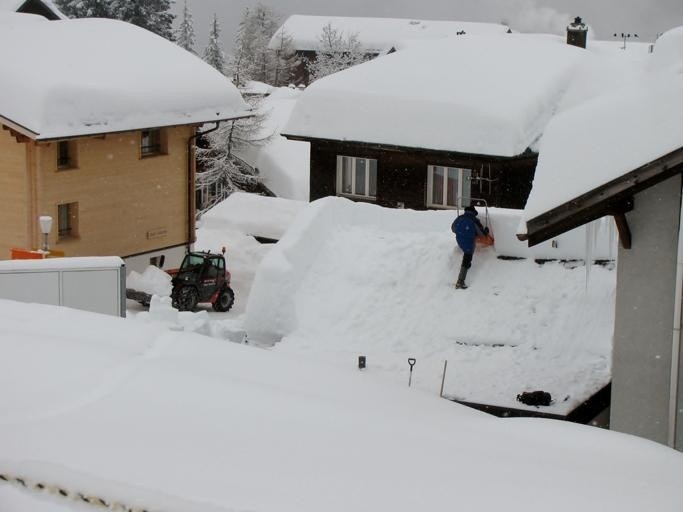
[484,227,489,236]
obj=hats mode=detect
[464,204,478,216]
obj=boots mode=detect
[454,267,468,289]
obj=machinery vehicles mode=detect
[124,244,237,315]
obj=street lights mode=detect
[38,215,54,259]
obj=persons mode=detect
[451,205,489,288]
